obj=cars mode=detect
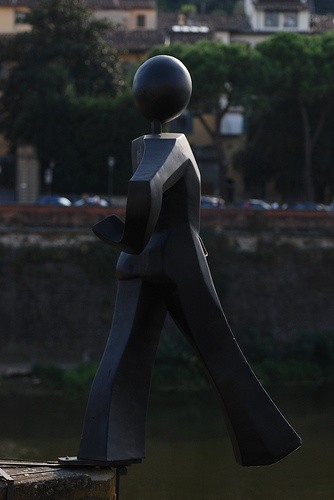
[36,195,111,208]
[198,191,334,212]
